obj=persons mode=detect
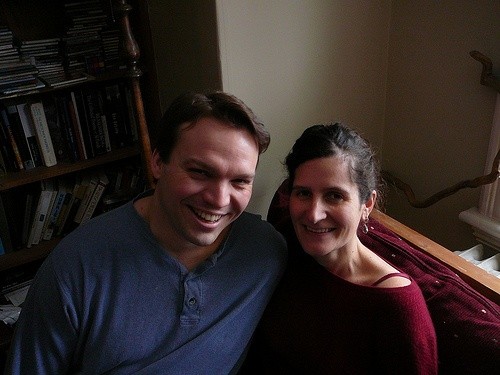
[236,123,438,375]
[3,91,288,375]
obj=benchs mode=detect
[267,179,500,375]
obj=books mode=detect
[0,0,150,257]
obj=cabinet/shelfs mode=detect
[0,0,163,352]
